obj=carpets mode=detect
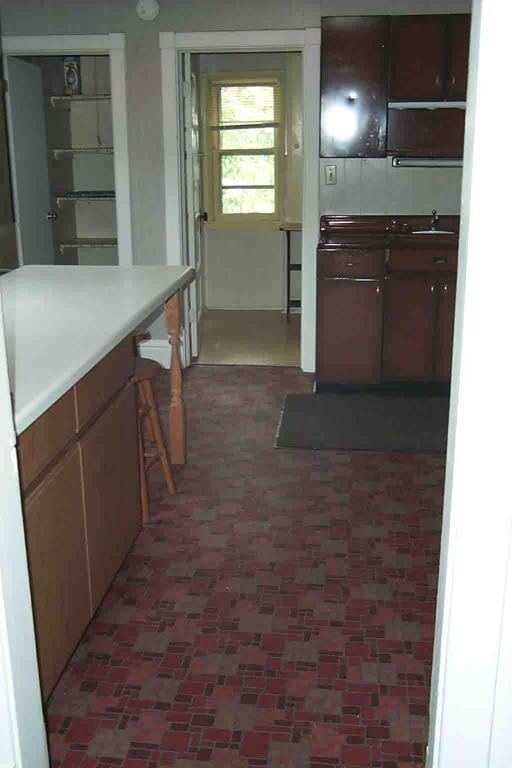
[267,389,449,455]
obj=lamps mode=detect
[392,156,463,167]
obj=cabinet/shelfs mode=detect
[313,246,389,390]
[384,11,476,158]
[381,243,459,386]
[317,14,389,159]
[49,93,117,250]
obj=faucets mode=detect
[431,209,440,231]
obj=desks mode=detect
[0,262,198,712]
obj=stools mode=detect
[275,219,307,320]
[131,355,180,529]
